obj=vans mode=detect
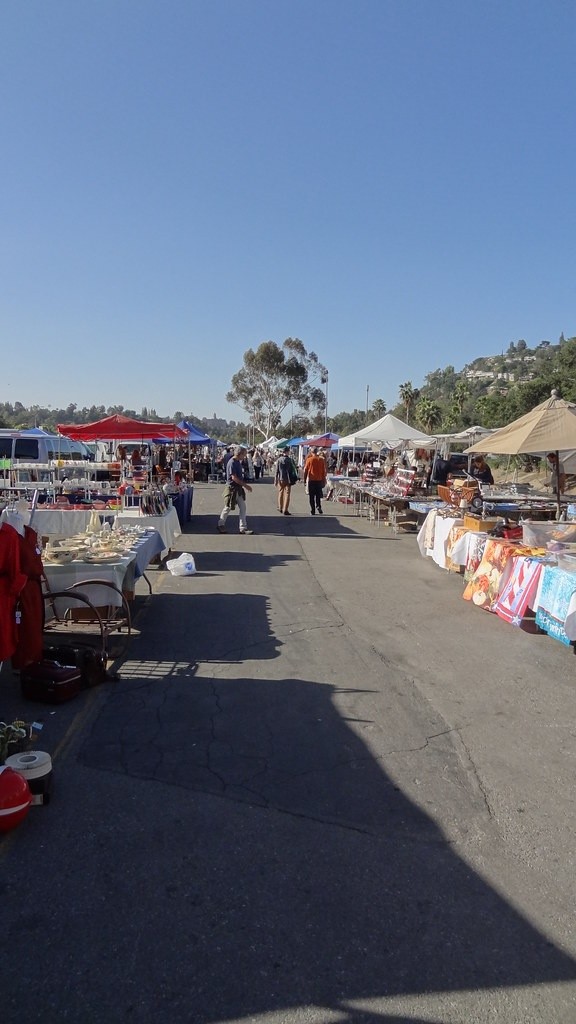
[0,434,152,502]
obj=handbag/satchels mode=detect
[166,553,196,576]
[290,458,298,482]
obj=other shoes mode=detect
[317,506,323,513]
[311,511,315,515]
[284,511,291,515]
[277,507,283,513]
[216,526,228,533]
[240,529,254,534]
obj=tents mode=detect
[57,413,228,494]
[335,416,504,472]
[203,431,341,451]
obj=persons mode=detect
[130,450,142,465]
[473,456,493,484]
[545,453,566,496]
[274,445,365,515]
[428,454,450,486]
[116,445,276,479]
[216,446,254,535]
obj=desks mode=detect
[325,473,576,646]
[0,485,193,562]
[41,528,167,633]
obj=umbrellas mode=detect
[463,389,575,506]
